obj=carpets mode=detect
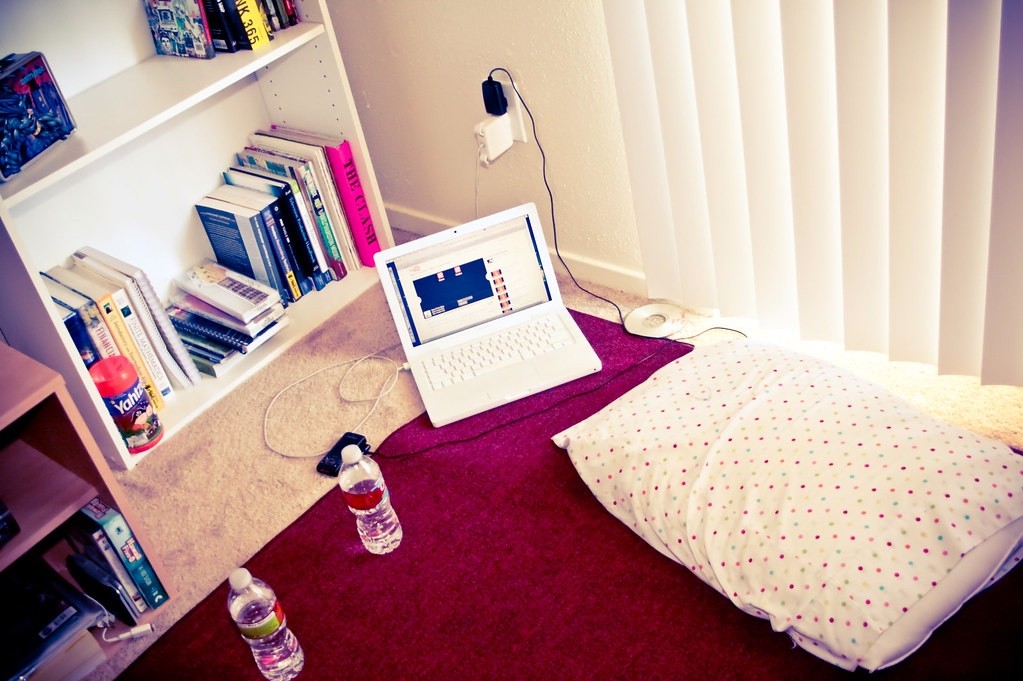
[109,307,1023,681]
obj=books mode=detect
[38,123,382,413]
[72,497,169,613]
[142,0,302,60]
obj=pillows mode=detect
[550,336,1023,673]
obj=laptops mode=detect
[372,203,603,426]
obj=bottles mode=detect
[339,444,403,555]
[227,567,305,681]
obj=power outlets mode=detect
[499,81,528,144]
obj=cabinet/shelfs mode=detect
[0,0,397,472]
[0,343,178,681]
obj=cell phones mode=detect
[315,432,367,478]
